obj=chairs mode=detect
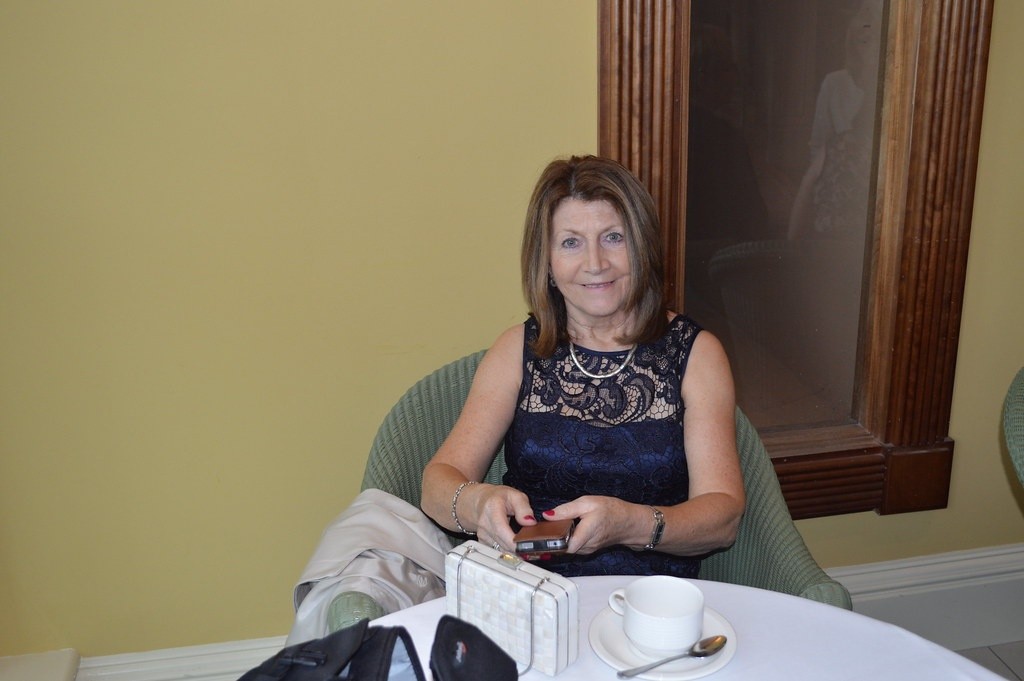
[319,343,851,636]
[998,363,1024,494]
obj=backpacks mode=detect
[440,538,582,676]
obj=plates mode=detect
[588,602,738,680]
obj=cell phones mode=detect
[512,520,576,554]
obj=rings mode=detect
[492,541,499,549]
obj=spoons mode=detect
[616,634,727,680]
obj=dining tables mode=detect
[231,577,1009,681]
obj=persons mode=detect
[784,8,879,239]
[420,152,747,579]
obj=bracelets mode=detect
[453,481,478,535]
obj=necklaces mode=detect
[566,328,638,379]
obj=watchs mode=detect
[632,501,664,552]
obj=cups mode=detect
[608,575,705,659]
